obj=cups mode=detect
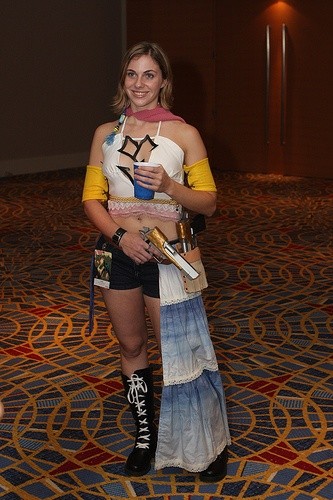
[133,162,159,200]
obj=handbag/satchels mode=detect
[174,237,208,293]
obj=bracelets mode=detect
[111,228,127,246]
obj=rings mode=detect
[147,245,151,250]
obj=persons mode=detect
[81,40,233,482]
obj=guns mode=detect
[141,226,198,282]
[178,219,202,255]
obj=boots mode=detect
[120,363,159,476]
[199,448,228,482]
